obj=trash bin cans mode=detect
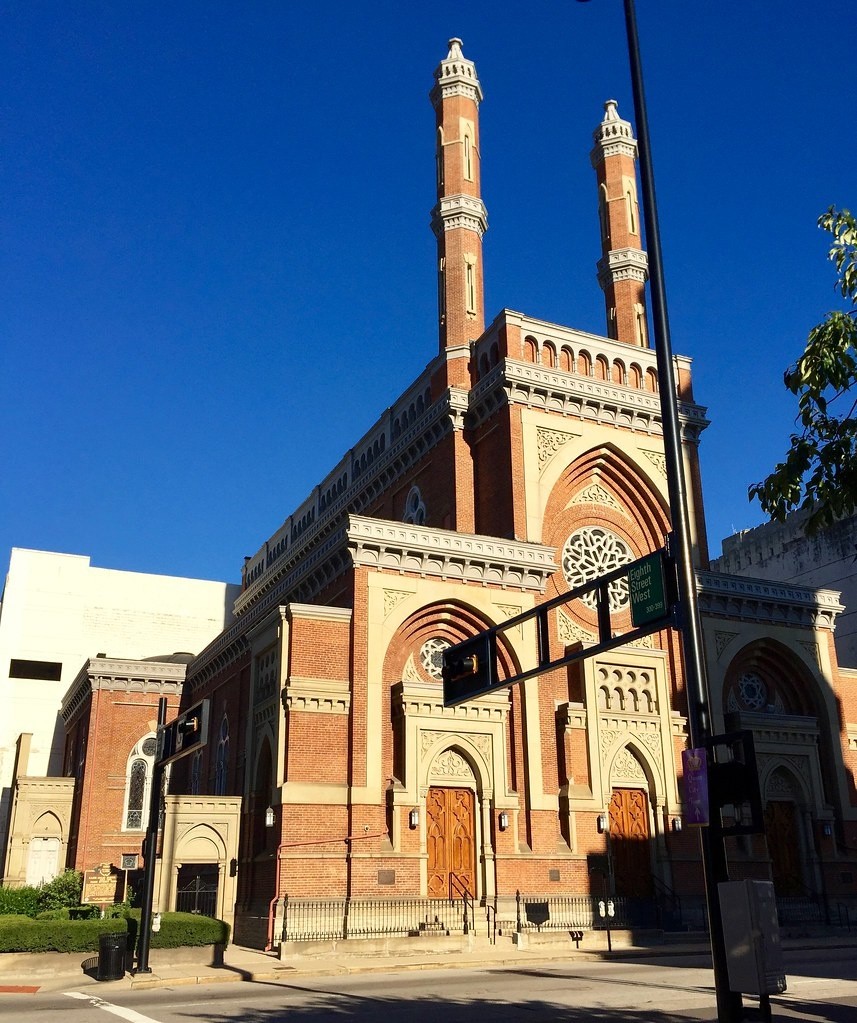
[97,932,130,981]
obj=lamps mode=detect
[412,806,419,826]
[502,812,508,827]
[675,817,681,830]
[266,806,276,826]
[600,815,606,829]
[824,823,832,836]
